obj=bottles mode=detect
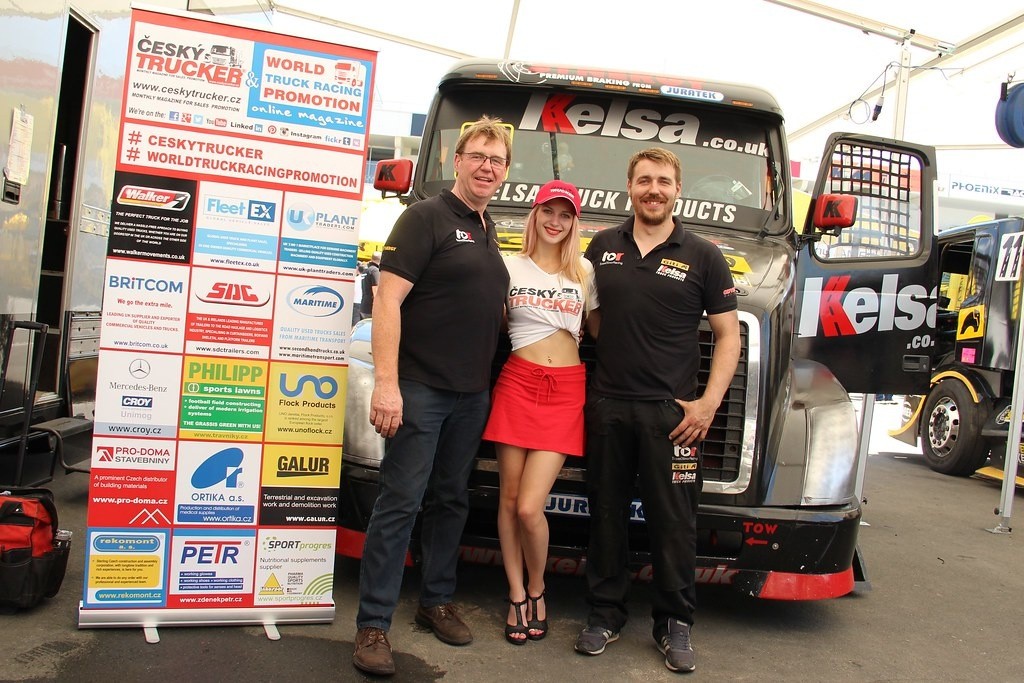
[54,529,73,549]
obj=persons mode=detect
[490,182,602,644]
[580,145,742,674]
[350,113,586,675]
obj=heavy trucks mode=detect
[890,219,1024,493]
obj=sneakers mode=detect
[353,627,396,676]
[652,616,696,672]
[573,622,620,655]
[416,603,472,646]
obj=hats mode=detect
[531,180,581,220]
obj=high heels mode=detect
[504,591,529,645]
[526,584,548,641]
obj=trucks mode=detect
[337,59,937,603]
[1,4,131,454]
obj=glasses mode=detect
[461,152,508,167]
